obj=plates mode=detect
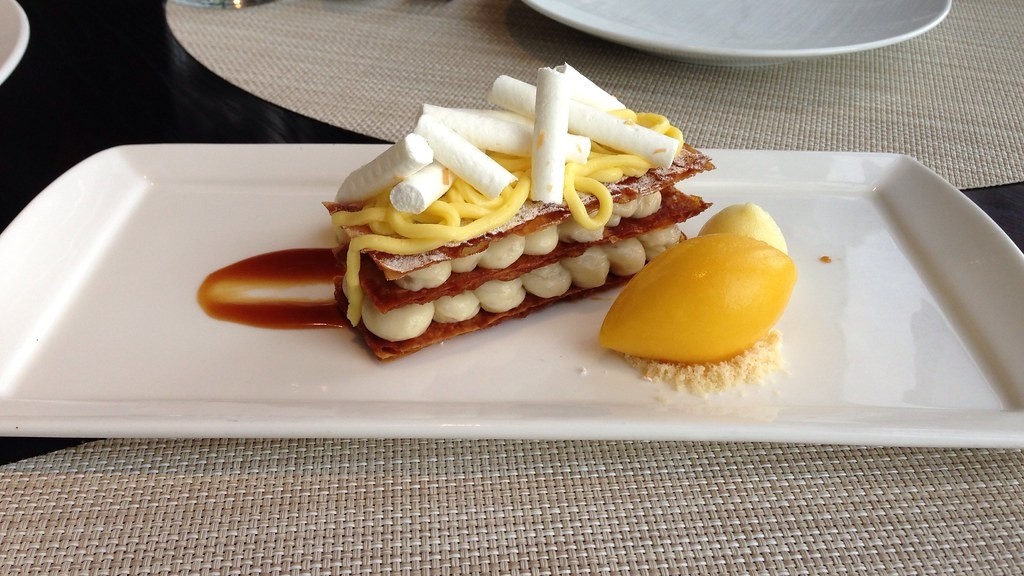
[521,0,953,64]
[0,142,1024,450]
[0,0,32,86]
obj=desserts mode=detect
[598,200,797,394]
[323,61,715,362]
